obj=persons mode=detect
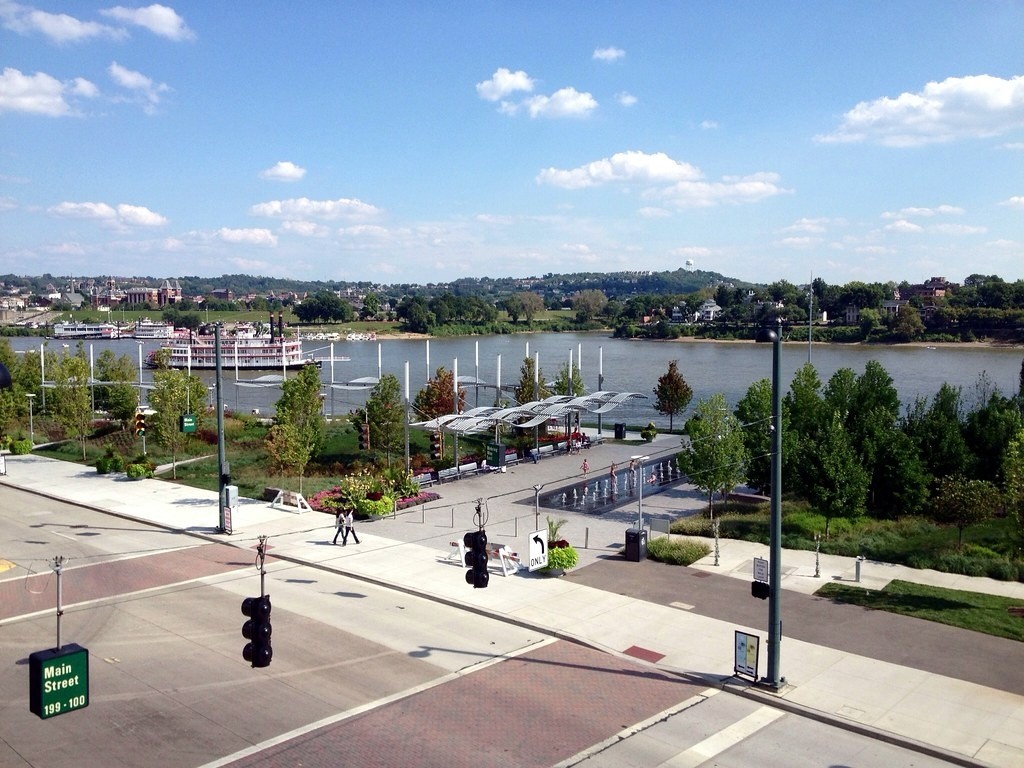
[629,459,637,476]
[564,437,573,457]
[647,472,658,485]
[330,507,347,545]
[341,507,363,547]
[579,458,590,479]
[570,427,582,444]
[583,437,589,445]
[523,442,541,464]
[582,432,590,449]
[610,460,618,482]
[575,438,581,455]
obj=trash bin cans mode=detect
[625,528,647,562]
[615,422,626,439]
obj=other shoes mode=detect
[332,541,337,545]
[343,540,346,546]
[356,541,362,544]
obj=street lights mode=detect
[632,454,650,530]
[756,318,783,688]
[25,393,36,445]
[199,322,229,531]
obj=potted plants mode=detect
[537,513,579,576]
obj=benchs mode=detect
[459,462,479,478]
[530,444,558,461]
[505,453,523,465]
[410,473,437,487]
[438,466,459,485]
[558,439,583,452]
[586,433,607,445]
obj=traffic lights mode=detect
[429,432,443,461]
[752,583,769,599]
[136,410,146,439]
[358,424,371,450]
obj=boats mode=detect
[143,329,323,370]
[46,319,132,341]
[130,318,190,339]
